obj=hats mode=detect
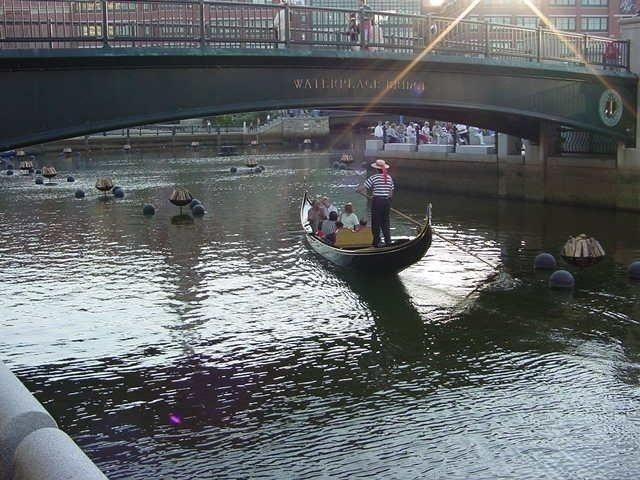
[371,159,390,170]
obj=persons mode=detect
[374,120,495,150]
[349,13,360,51]
[358,0,374,51]
[605,41,619,70]
[307,196,360,245]
[273,3,292,49]
[355,159,395,248]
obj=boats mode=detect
[219,144,239,156]
[300,190,433,271]
